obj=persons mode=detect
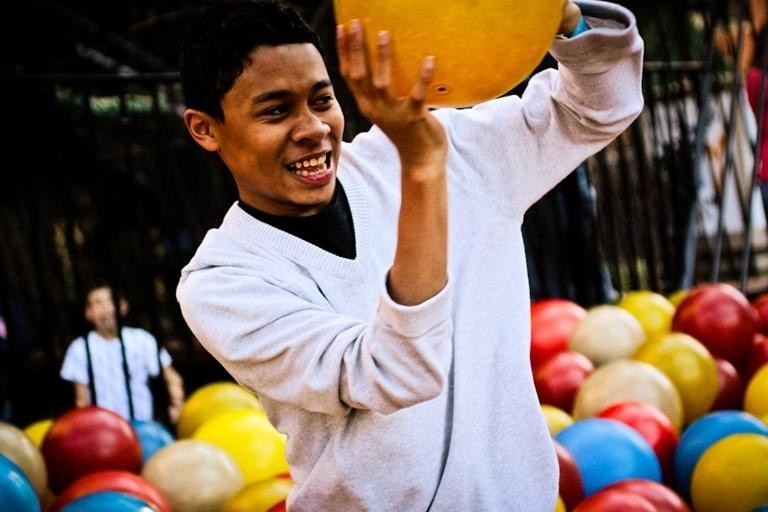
[175,0,645,512]
[59,282,186,425]
